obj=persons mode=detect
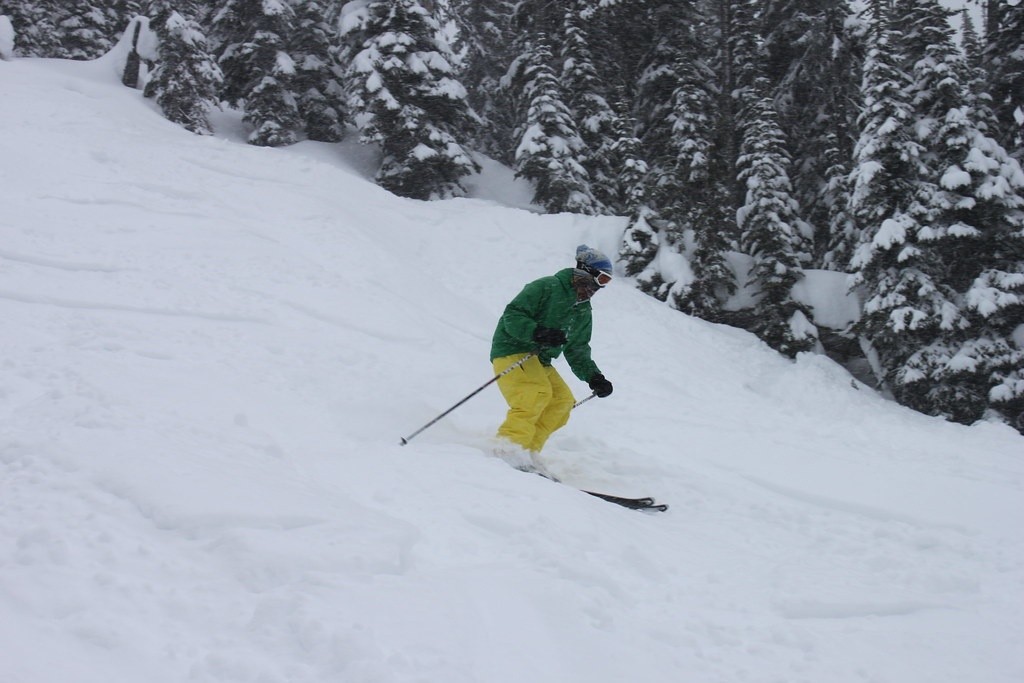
[489,244,614,475]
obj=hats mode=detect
[575,244,612,276]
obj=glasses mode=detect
[594,270,613,286]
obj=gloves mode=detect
[588,374,613,398]
[533,324,568,347]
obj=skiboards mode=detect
[516,465,670,514]
